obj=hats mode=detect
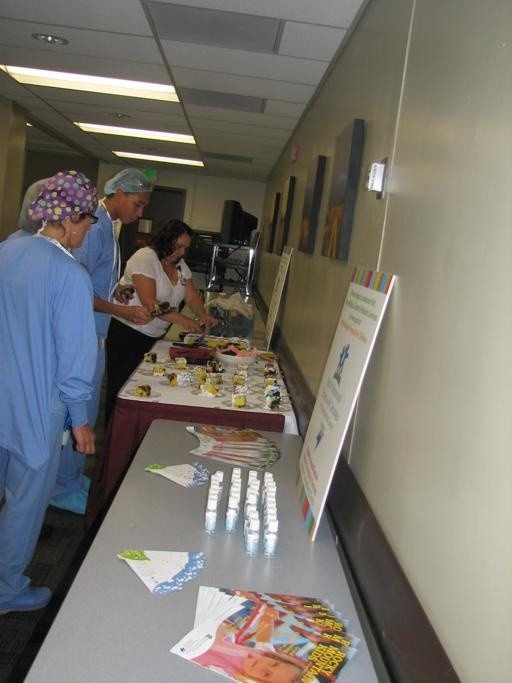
[28,169,98,221]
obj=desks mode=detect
[162,291,273,350]
[25,419,392,683]
[86,338,300,534]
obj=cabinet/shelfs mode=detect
[206,243,258,297]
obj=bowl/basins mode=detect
[216,349,258,367]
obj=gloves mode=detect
[114,283,137,305]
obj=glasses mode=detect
[80,212,99,224]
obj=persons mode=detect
[0,170,99,616]
[4,178,71,541]
[105,220,221,431]
[49,169,153,516]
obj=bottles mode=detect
[204,466,242,535]
[245,469,280,558]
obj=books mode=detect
[168,586,361,683]
[186,425,282,471]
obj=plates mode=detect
[123,354,294,416]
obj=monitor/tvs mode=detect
[221,200,258,245]
